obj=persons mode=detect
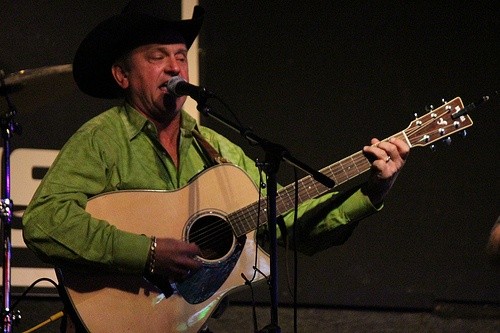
[22,0,409,333]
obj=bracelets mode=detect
[148,235,157,273]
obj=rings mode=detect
[385,155,391,163]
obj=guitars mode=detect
[54,91,500,333]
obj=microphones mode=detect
[166,76,211,101]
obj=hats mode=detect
[73,5,203,98]
